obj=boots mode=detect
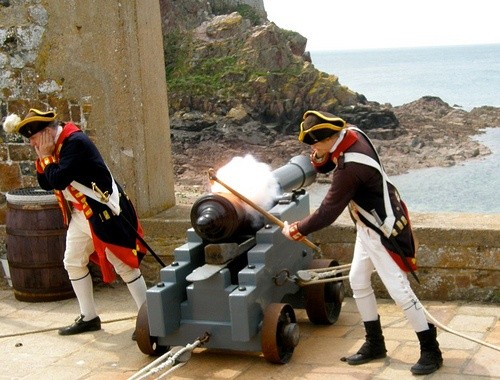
[347,314,387,364]
[411,323,443,374]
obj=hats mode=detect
[3,109,56,139]
[299,110,346,145]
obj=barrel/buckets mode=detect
[6,186,89,303]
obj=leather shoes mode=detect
[58,315,101,335]
[132,330,137,340]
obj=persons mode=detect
[280,110,442,374]
[13,107,147,335]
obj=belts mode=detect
[71,202,83,210]
